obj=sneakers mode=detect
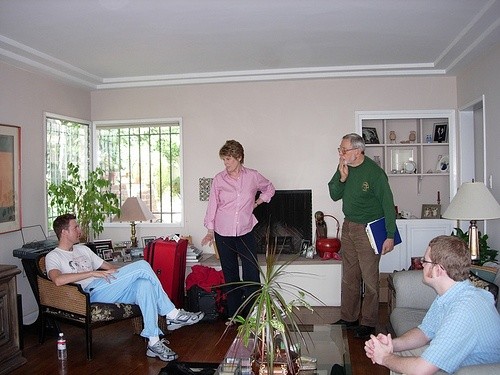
[166,309,204,330]
[146,340,179,361]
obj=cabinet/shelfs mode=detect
[0,264,27,375]
[355,110,457,274]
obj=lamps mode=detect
[441,181,500,264]
[110,196,157,248]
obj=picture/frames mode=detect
[362,127,380,144]
[141,236,156,248]
[0,123,23,236]
[432,122,449,143]
[122,254,133,263]
[93,240,113,259]
[421,204,441,219]
[101,248,114,262]
[299,239,311,257]
[111,251,122,261]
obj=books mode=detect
[217,335,317,375]
[186,245,203,263]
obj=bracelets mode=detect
[208,232,213,234]
[255,201,259,206]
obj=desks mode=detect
[186,254,342,307]
[387,272,396,315]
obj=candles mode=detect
[438,191,440,201]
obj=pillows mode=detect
[469,264,498,282]
[468,270,500,309]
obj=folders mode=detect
[364,217,402,255]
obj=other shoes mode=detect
[330,319,359,329]
[351,324,376,337]
[225,318,241,325]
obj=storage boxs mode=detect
[187,285,220,323]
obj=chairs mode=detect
[20,243,149,361]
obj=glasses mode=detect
[337,148,358,154]
[420,257,444,270]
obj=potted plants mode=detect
[205,213,329,375]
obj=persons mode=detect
[201,140,275,321]
[328,133,396,340]
[364,235,500,375]
[45,214,204,361]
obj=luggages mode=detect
[144,238,188,308]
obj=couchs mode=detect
[386,262,500,375]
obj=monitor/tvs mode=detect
[253,190,312,254]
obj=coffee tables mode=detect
[214,324,352,375]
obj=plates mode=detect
[404,161,416,173]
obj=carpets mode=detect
[157,361,353,375]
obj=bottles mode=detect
[57,333,67,361]
[373,156,381,165]
